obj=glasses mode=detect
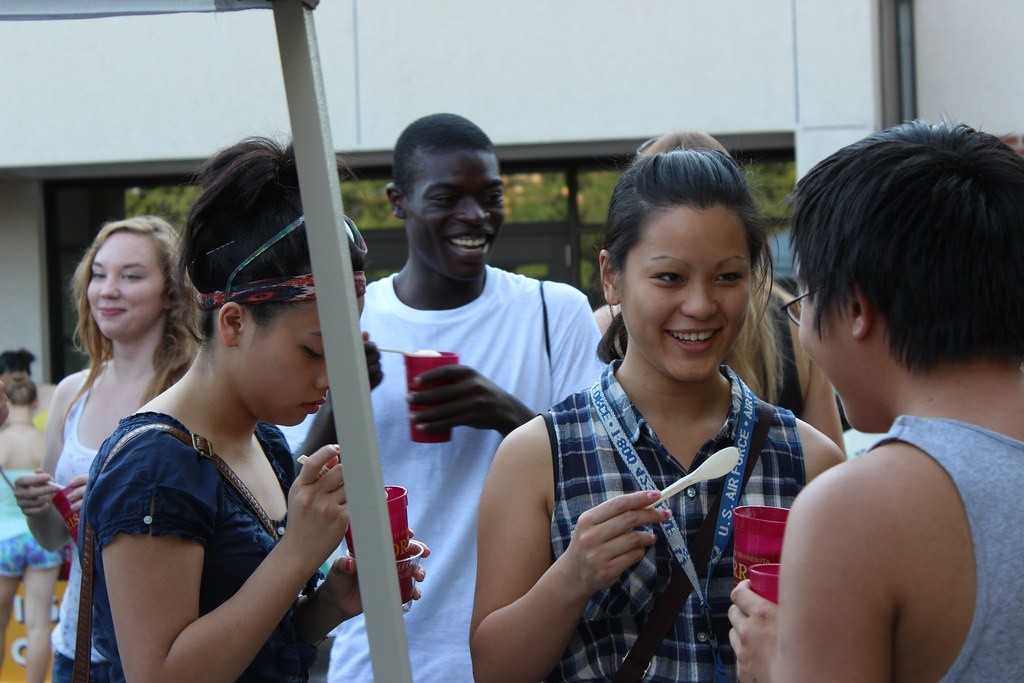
[224,213,369,303]
[780,283,834,326]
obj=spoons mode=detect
[377,348,442,357]
[643,447,740,510]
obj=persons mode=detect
[1,113,847,683]
[726,110,1024,683]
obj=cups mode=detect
[347,541,424,614]
[405,352,459,443]
[732,505,791,588]
[345,486,413,604]
[51,486,80,547]
[749,564,780,605]
